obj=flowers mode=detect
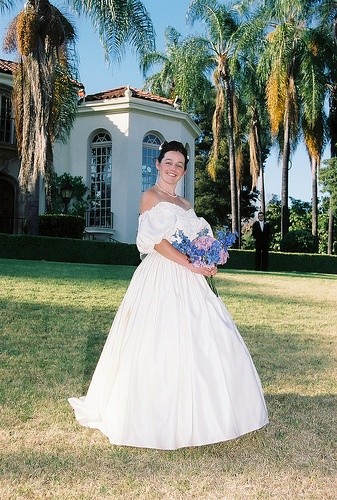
[171,225,238,297]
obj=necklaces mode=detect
[155,182,178,199]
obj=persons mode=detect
[252,212,271,271]
[67,141,270,450]
